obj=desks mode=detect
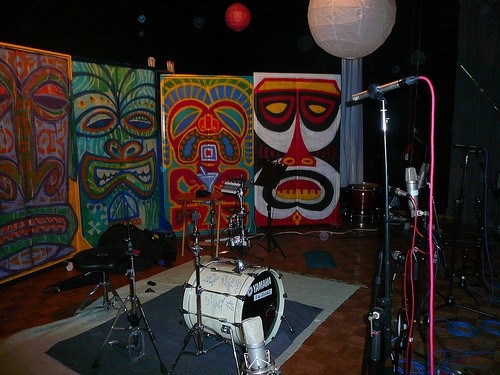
[175,192,225,257]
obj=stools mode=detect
[72,248,126,316]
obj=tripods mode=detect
[73,162,288,375]
[362,102,500,375]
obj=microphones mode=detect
[418,162,429,190]
[241,316,279,375]
[388,185,411,199]
[405,167,418,217]
[351,76,417,102]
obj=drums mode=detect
[182,258,285,348]
[344,183,387,222]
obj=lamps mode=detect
[225,2,252,31]
[307,0,397,60]
